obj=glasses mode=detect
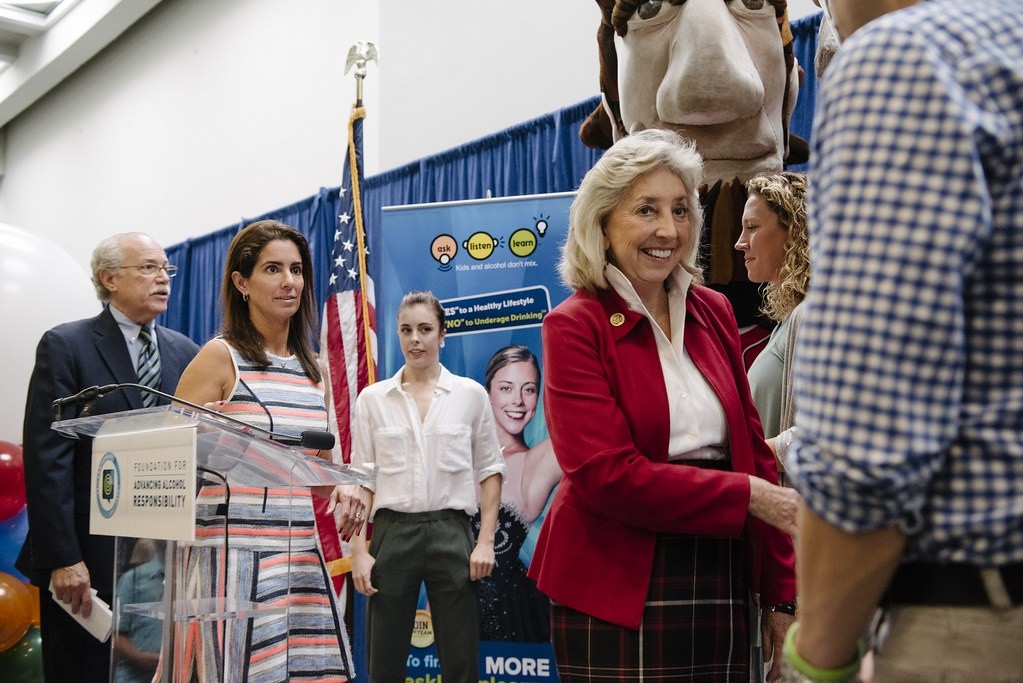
[109,264,179,278]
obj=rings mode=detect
[355,513,362,521]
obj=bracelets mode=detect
[770,602,796,616]
[783,619,865,683]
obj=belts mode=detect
[878,559,1023,609]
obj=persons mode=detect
[525,128,800,683]
[109,537,168,683]
[16,231,203,683]
[734,170,812,493]
[150,220,367,683]
[786,0,1023,683]
[351,290,508,683]
[468,343,565,644]
[580,0,811,381]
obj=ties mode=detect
[137,326,161,409]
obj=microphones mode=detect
[79,383,335,450]
[52,385,99,421]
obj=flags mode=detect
[311,100,381,603]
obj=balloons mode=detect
[0,438,45,683]
